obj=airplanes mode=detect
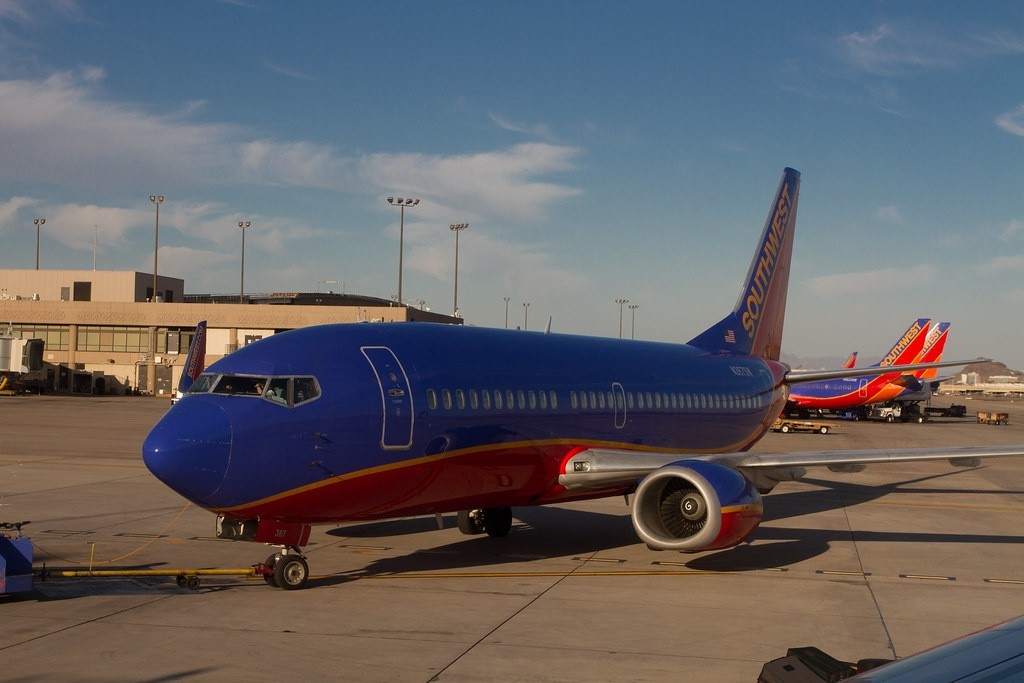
[142,166,1024,591]
[778,317,952,421]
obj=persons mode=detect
[779,412,786,421]
[122,376,130,396]
[816,410,821,419]
[146,297,150,302]
[224,379,307,405]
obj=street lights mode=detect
[628,305,639,340]
[449,222,469,318]
[238,221,251,306]
[614,298,629,339]
[386,197,420,307]
[523,302,530,330]
[148,194,164,302]
[503,297,510,329]
[34,219,46,270]
